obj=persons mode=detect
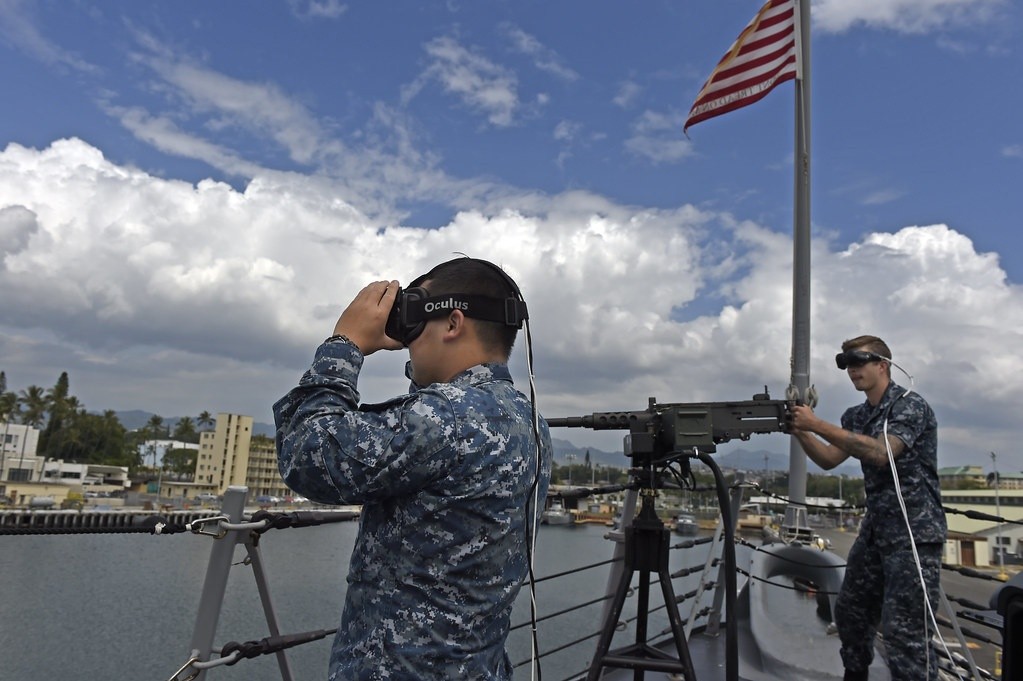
[274,257,554,681]
[783,336,948,681]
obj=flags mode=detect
[683,0,803,141]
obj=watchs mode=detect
[324,333,360,350]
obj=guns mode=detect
[544,383,802,468]
